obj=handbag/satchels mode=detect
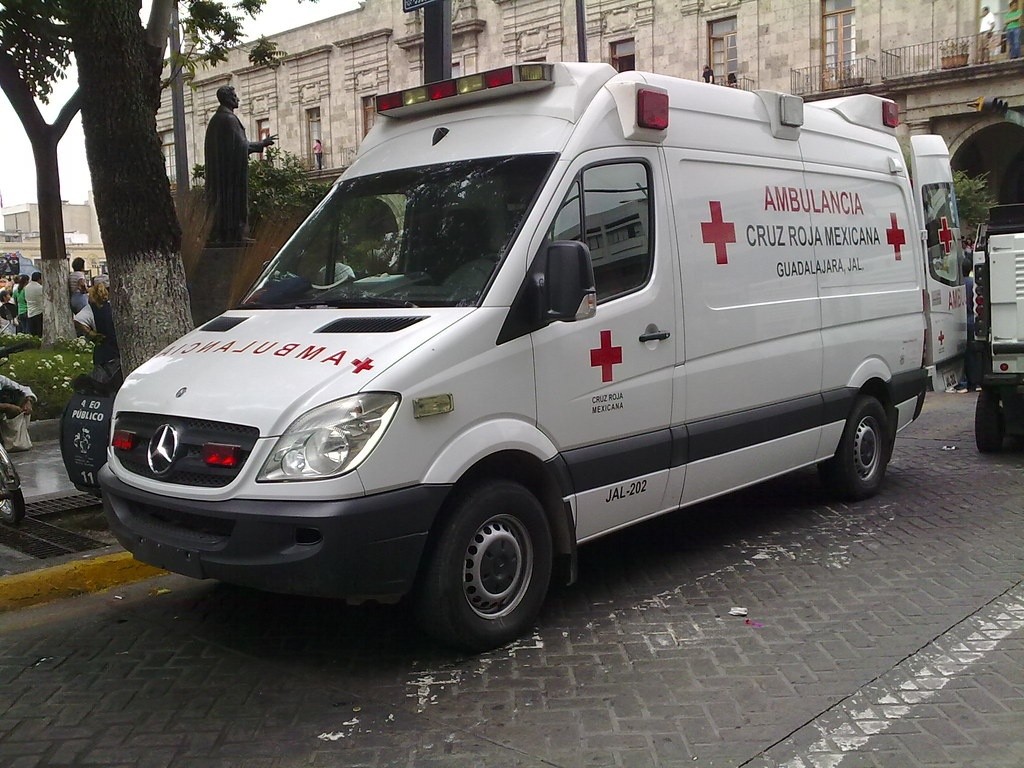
[0,409,33,453]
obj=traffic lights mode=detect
[976,99,1009,115]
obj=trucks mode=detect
[972,205,1023,451]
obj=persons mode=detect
[0,251,110,337]
[1002,0,1023,59]
[979,7,995,64]
[202,85,279,246]
[0,374,37,448]
[313,139,323,170]
[947,234,982,394]
[702,64,714,84]
[74,282,120,368]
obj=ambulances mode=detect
[94,58,969,656]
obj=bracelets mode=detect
[25,397,34,405]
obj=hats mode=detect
[94,274,111,289]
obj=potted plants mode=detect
[838,65,865,88]
[939,39,969,68]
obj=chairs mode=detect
[439,259,496,305]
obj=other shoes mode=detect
[945,383,968,394]
[976,384,983,392]
